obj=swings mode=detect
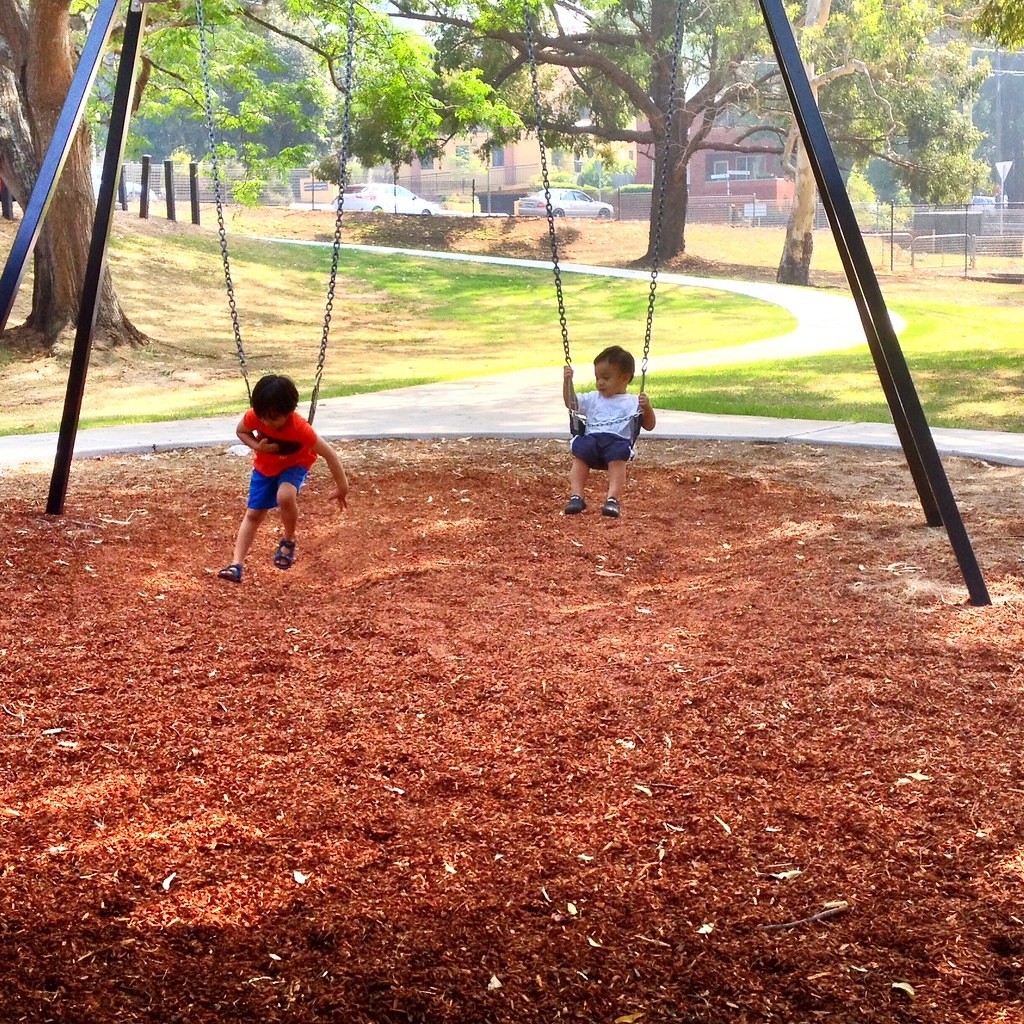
[522,0,684,470]
[195,0,355,455]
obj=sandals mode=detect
[274,537,295,571]
[218,564,242,583]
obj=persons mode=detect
[218,375,350,585]
[562,346,655,517]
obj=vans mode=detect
[439,192,482,215]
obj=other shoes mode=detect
[565,495,586,514]
[602,497,619,516]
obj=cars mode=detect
[519,188,616,220]
[330,183,439,217]
